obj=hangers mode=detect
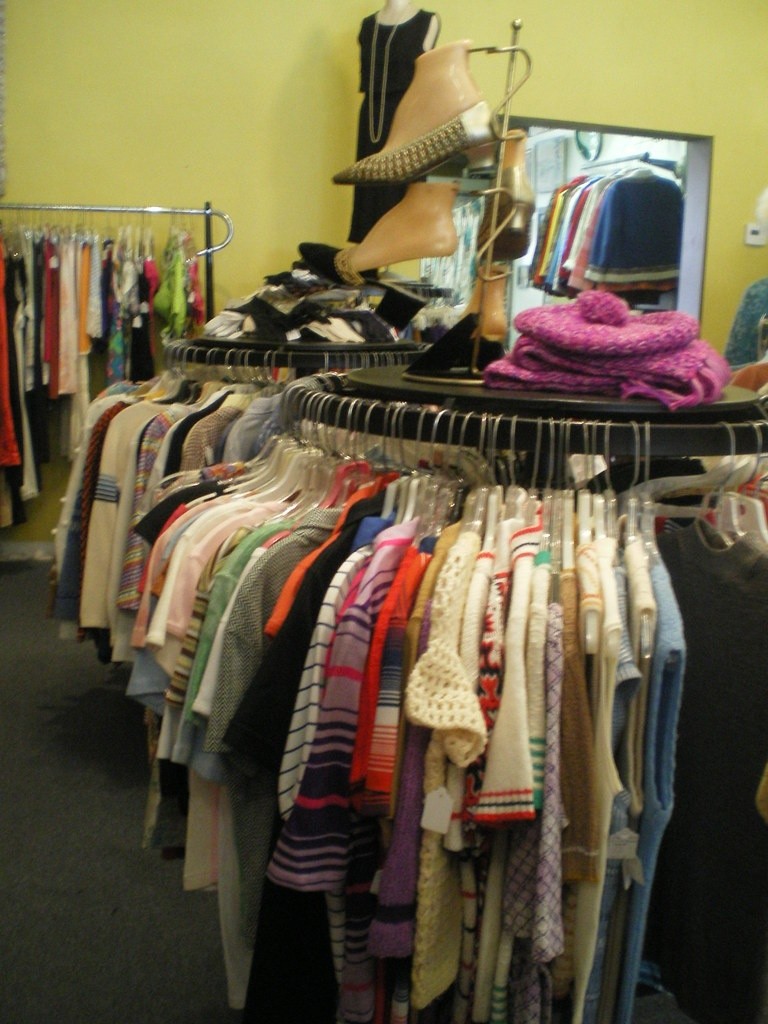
[160,338,768,571]
[5,206,196,253]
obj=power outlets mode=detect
[746,223,766,244]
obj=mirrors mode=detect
[499,115,715,345]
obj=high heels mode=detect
[476,165,536,260]
[298,242,429,331]
[330,101,497,185]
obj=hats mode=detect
[514,290,701,356]
